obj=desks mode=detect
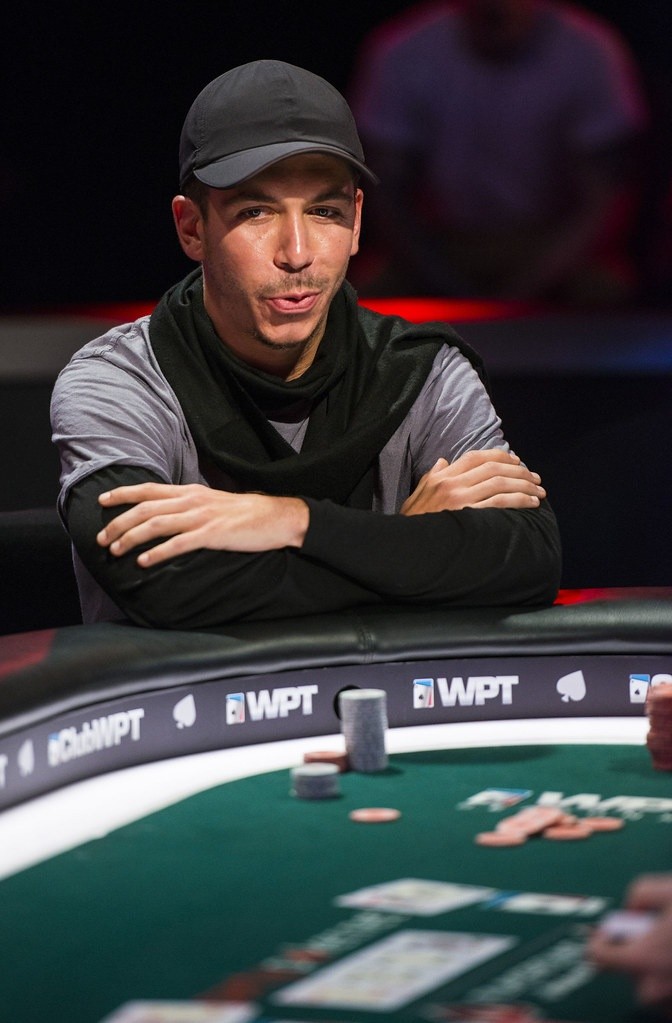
[0,595,672,1023]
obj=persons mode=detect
[334,0,647,332]
[45,56,568,637]
[584,873,671,1006]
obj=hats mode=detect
[179,60,378,190]
